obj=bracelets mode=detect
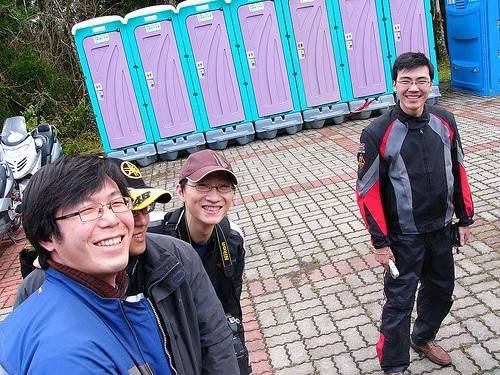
[371,247,391,255]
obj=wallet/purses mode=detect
[451,222,461,253]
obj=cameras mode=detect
[388,259,399,279]
[226,313,245,361]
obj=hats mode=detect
[106,158,172,210]
[178,149,241,185]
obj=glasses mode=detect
[130,202,157,217]
[54,196,133,223]
[395,80,432,86]
[185,182,237,193]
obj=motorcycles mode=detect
[0,116,63,246]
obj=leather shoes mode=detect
[387,371,404,375]
[410,337,451,365]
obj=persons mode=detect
[12,157,243,375]
[0,153,179,375]
[147,149,252,375]
[356,51,476,375]
